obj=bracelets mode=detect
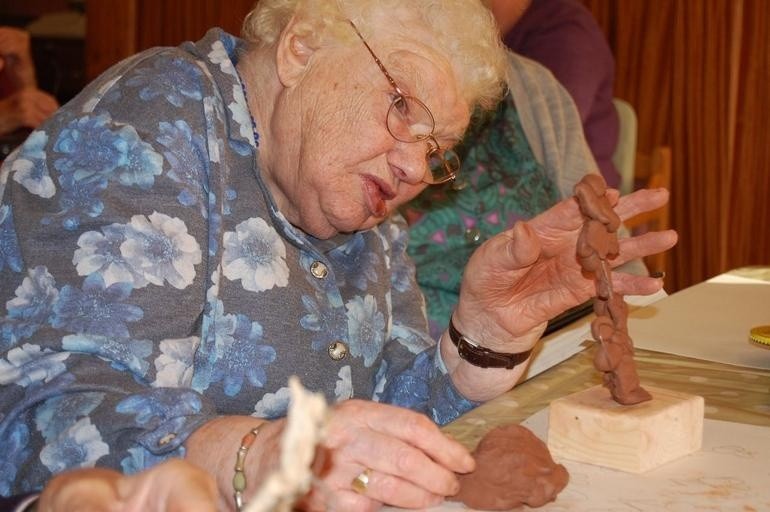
[443,313,531,369]
[234,418,274,512]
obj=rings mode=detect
[350,469,373,494]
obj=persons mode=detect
[393,0,673,381]
[0,26,61,163]
[503,1,620,188]
[0,0,683,512]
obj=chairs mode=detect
[517,96,677,384]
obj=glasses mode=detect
[344,18,463,187]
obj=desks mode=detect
[382,263,770,511]
